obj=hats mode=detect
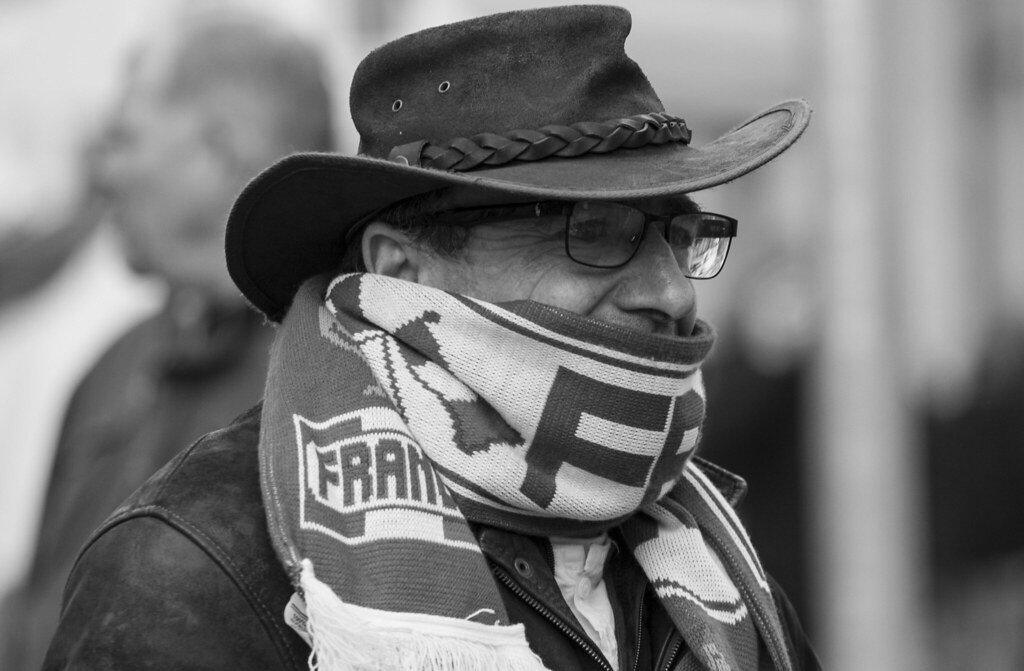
[226,5,811,323]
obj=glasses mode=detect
[400,199,738,280]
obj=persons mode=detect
[0,1,337,671]
[42,3,827,671]
